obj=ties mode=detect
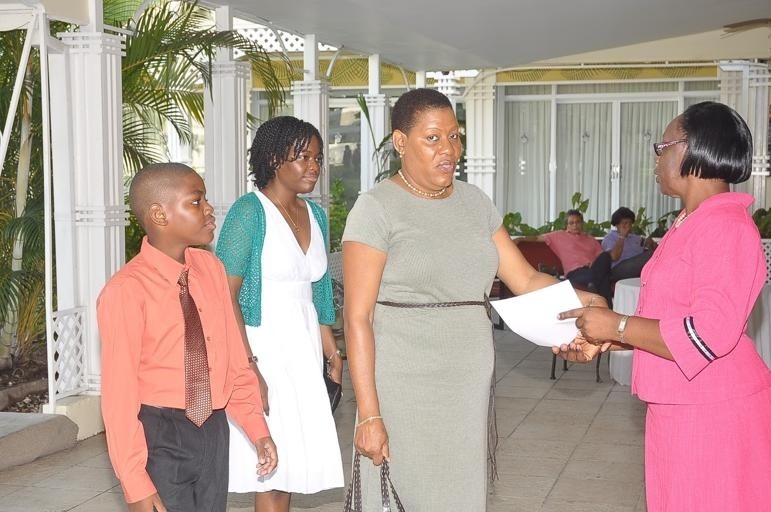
[177,269,213,428]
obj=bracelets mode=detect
[356,413,384,428]
[618,314,629,347]
[325,351,343,372]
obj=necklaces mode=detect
[397,170,446,202]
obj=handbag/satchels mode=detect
[325,380,342,418]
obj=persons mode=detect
[600,207,654,277]
[511,211,608,291]
[213,115,343,510]
[341,87,613,512]
[95,163,277,512]
[552,100,770,512]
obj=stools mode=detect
[551,353,603,383]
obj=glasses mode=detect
[654,139,687,156]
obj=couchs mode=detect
[499,239,604,330]
